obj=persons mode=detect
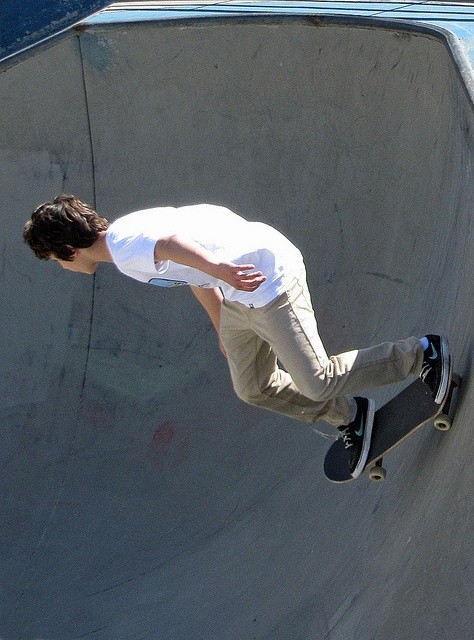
[22,192,449,481]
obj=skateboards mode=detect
[322,352,462,485]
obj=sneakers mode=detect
[420,333,448,404]
[337,398,374,478]
